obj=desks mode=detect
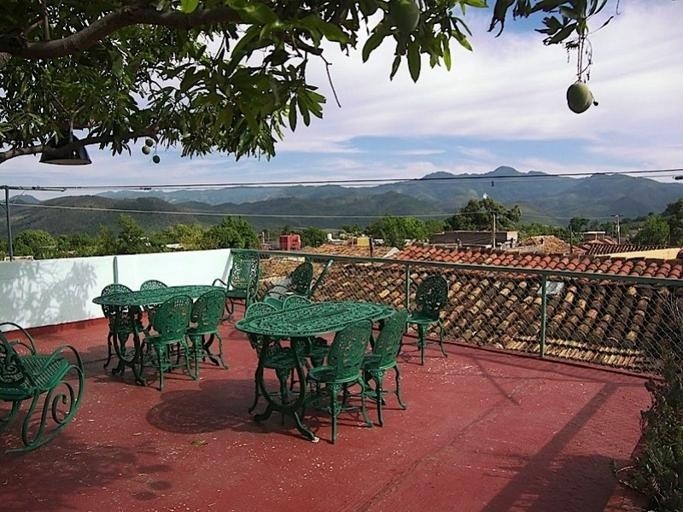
[92,284,225,385]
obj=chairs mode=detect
[93,279,229,390]
[213,251,260,318]
[0,321,87,456]
[404,276,449,365]
[235,258,406,441]
